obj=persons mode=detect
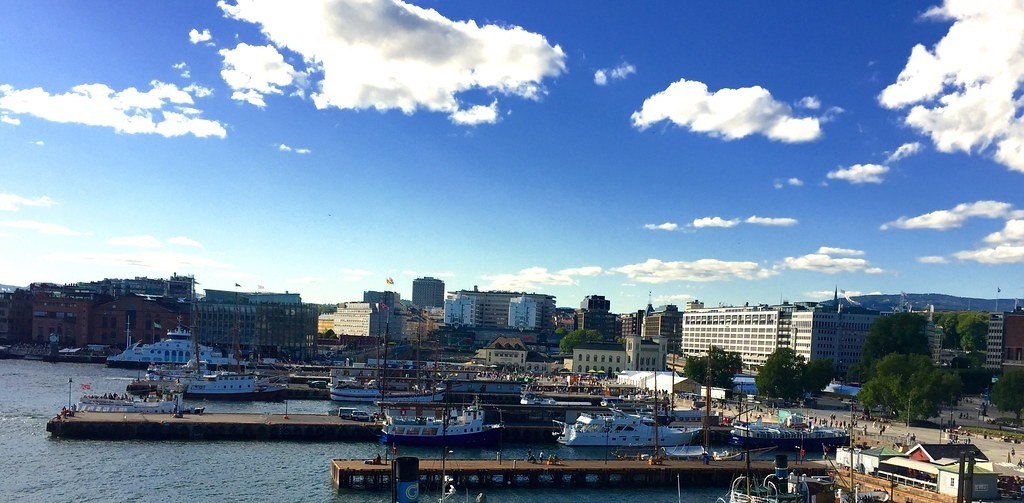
[657,389,668,396]
[480,369,562,380]
[56,404,76,420]
[376,453,381,463]
[1017,457,1023,468]
[1011,447,1015,457]
[526,449,559,465]
[83,392,134,401]
[949,433,971,445]
[910,432,916,442]
[814,410,894,435]
[959,412,969,419]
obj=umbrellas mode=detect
[587,368,619,378]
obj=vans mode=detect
[338,408,358,419]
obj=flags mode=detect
[380,302,388,310]
[386,278,394,284]
[997,287,1002,293]
[82,383,90,391]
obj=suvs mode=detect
[351,411,369,421]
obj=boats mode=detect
[68,314,890,503]
